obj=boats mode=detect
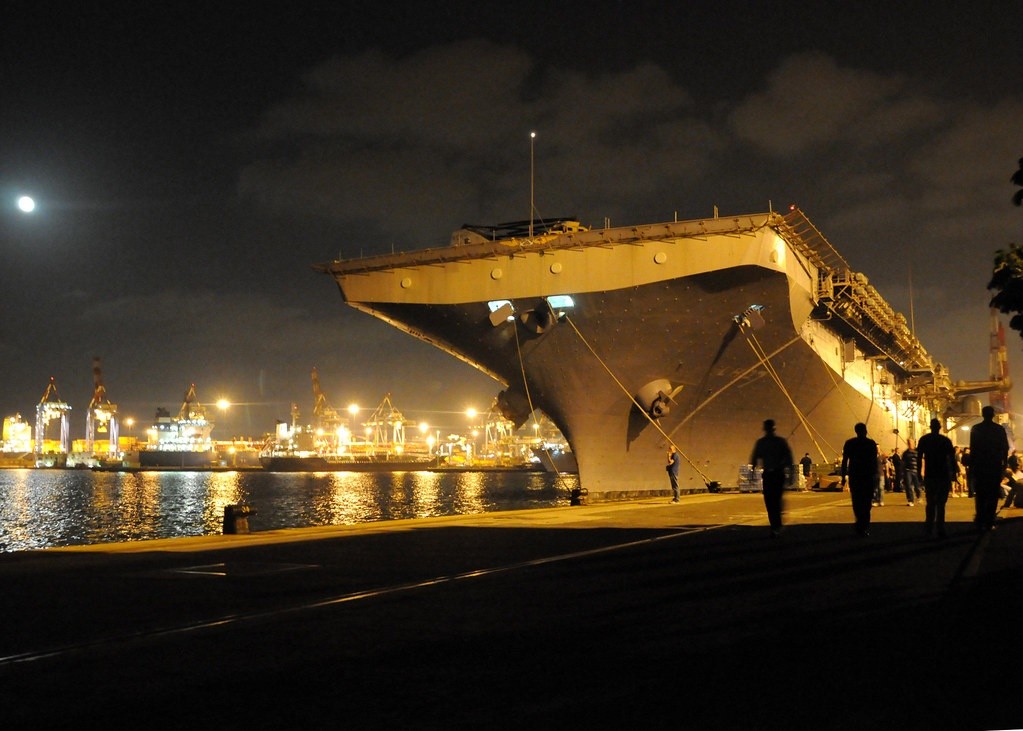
[529,446,579,472]
[256,364,442,473]
[309,131,958,504]
[138,382,216,467]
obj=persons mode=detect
[840,406,1023,524]
[666,445,680,504]
[750,419,795,525]
[799,453,812,477]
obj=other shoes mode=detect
[907,501,915,507]
[872,501,885,507]
[960,493,968,497]
[951,493,960,498]
[668,500,680,504]
